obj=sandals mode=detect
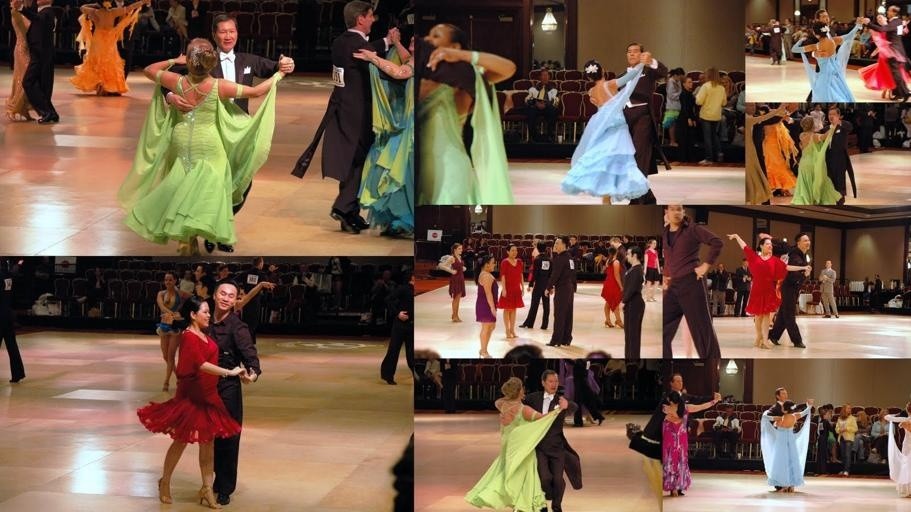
[163,382,169,392]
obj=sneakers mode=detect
[839,471,848,476]
[646,297,656,302]
[881,459,887,464]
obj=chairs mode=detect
[796,280,860,314]
[463,233,663,271]
[687,403,905,464]
[453,360,641,402]
[495,67,745,145]
[724,288,739,317]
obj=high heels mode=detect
[479,350,492,358]
[38,111,59,123]
[158,477,172,504]
[672,488,678,497]
[599,416,604,425]
[330,207,370,234]
[177,239,199,255]
[605,320,624,328]
[519,323,533,328]
[198,485,222,509]
[546,341,570,346]
[5,110,35,121]
[778,487,794,493]
[586,416,595,424]
[572,423,583,427]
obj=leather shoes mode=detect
[205,238,234,253]
[213,481,230,504]
[794,343,805,348]
[10,374,25,382]
[679,489,684,495]
[768,333,781,345]
[903,92,911,102]
[822,314,839,318]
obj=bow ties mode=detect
[219,52,236,62]
[544,392,554,401]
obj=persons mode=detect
[114,37,290,253]
[76,267,108,314]
[293,2,911,512]
[161,13,295,255]
[138,257,282,508]
[6,0,206,124]
[0,261,25,383]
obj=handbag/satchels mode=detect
[867,453,880,464]
